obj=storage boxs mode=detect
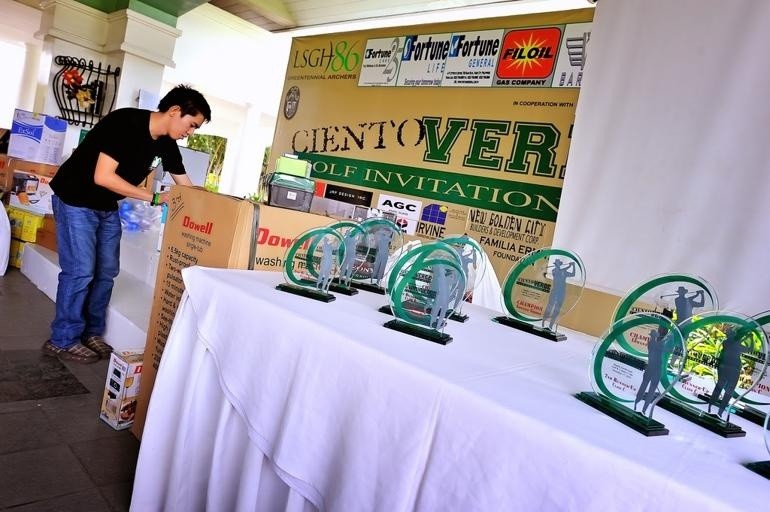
[127,182,347,446]
[0,106,68,270]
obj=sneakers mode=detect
[41,336,113,364]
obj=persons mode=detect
[43,83,211,364]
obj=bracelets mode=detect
[151,194,156,206]
[154,193,160,206]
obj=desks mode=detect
[169,264,770,512]
